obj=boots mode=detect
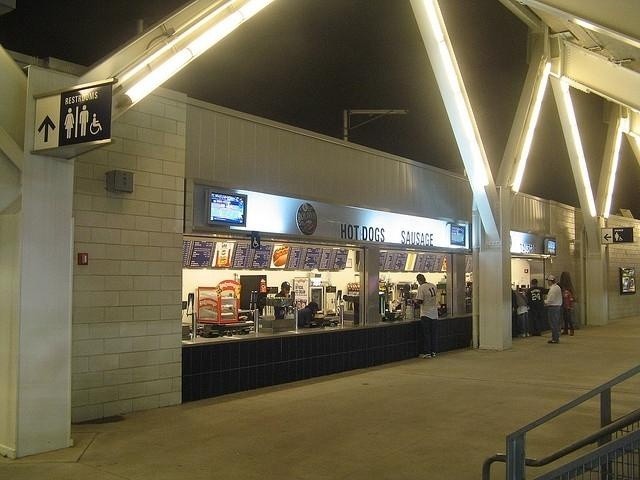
[561,328,574,336]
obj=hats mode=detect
[281,281,291,287]
[545,274,555,280]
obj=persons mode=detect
[466,283,473,297]
[275,281,293,319]
[298,302,319,327]
[511,272,577,344]
[413,274,441,360]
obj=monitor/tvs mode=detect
[207,189,247,226]
[544,239,557,256]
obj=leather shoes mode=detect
[548,340,560,344]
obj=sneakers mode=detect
[418,352,437,358]
